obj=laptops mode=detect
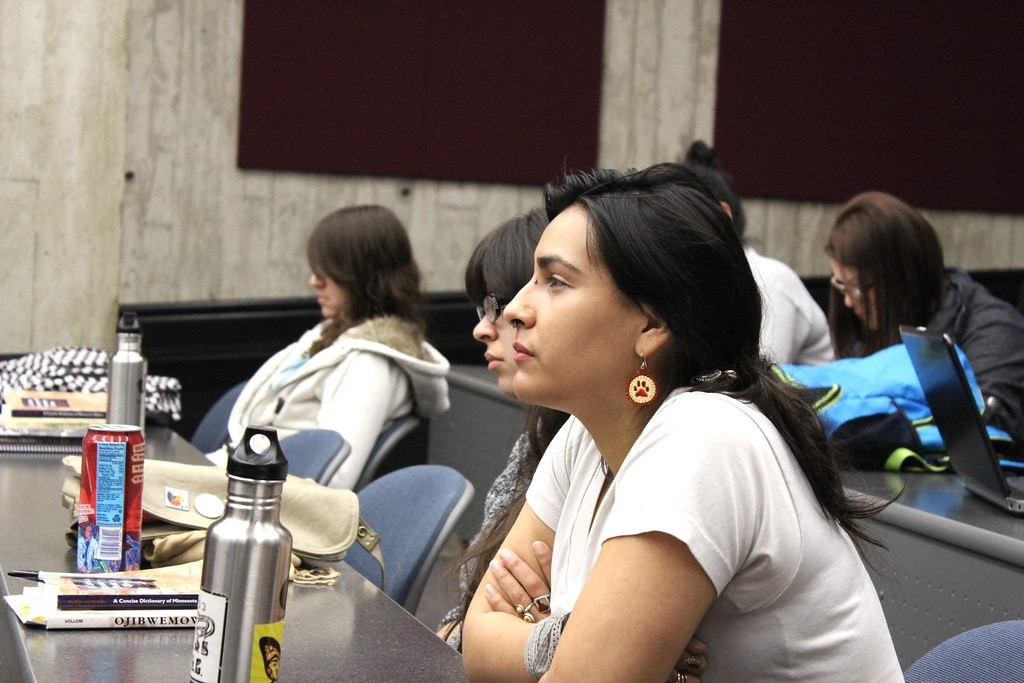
[899,325,1024,513]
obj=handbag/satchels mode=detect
[0,345,184,427]
[58,453,385,585]
[770,324,1022,476]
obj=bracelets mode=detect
[524,611,569,681]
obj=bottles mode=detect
[106,311,148,438]
[189,427,293,683]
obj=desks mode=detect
[424,364,1023,673]
[2,349,489,683]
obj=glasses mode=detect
[828,275,878,303]
[476,294,510,325]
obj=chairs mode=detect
[187,378,1024,683]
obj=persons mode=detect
[679,137,834,363]
[204,205,452,488]
[433,165,908,683]
[75,523,100,572]
[826,189,1024,465]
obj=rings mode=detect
[533,594,551,614]
[515,601,536,623]
[680,652,705,676]
[674,670,688,683]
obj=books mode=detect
[0,388,108,454]
[16,558,210,630]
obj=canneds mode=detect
[77,425,145,574]
